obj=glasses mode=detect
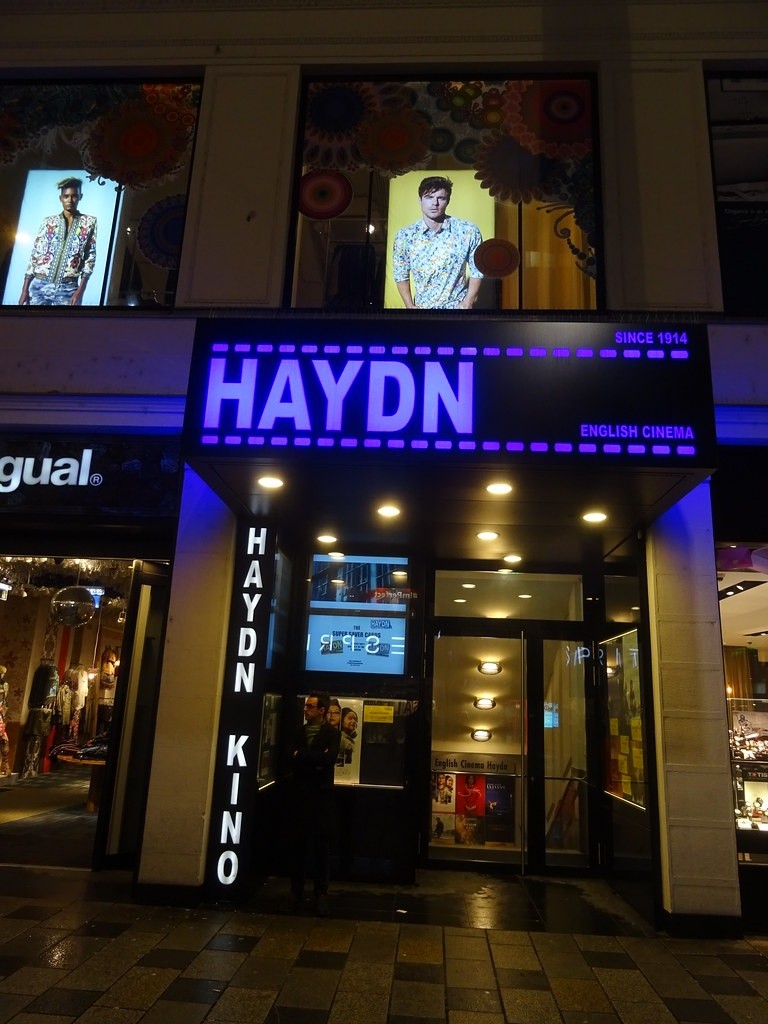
[327,710,341,716]
[304,703,317,710]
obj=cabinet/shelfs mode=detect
[727,697,768,865]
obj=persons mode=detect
[392,177,486,309]
[18,180,98,306]
[435,817,445,838]
[278,693,341,912]
[0,665,11,777]
[327,698,358,764]
[463,775,481,816]
[435,774,453,805]
[18,663,59,781]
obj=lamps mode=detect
[471,730,493,743]
[478,661,502,675]
[473,697,496,711]
[118,612,126,623]
[50,586,96,628]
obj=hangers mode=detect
[69,662,84,674]
[39,657,56,668]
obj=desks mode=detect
[57,753,105,814]
